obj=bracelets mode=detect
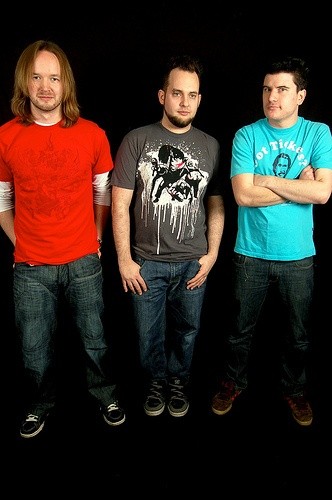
[97,233,103,243]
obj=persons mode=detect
[0,41,126,438]
[111,58,225,417]
[211,57,332,426]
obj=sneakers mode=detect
[20,410,51,438]
[212,387,242,415]
[166,380,190,417]
[101,401,125,426]
[285,396,312,425]
[144,386,165,416]
